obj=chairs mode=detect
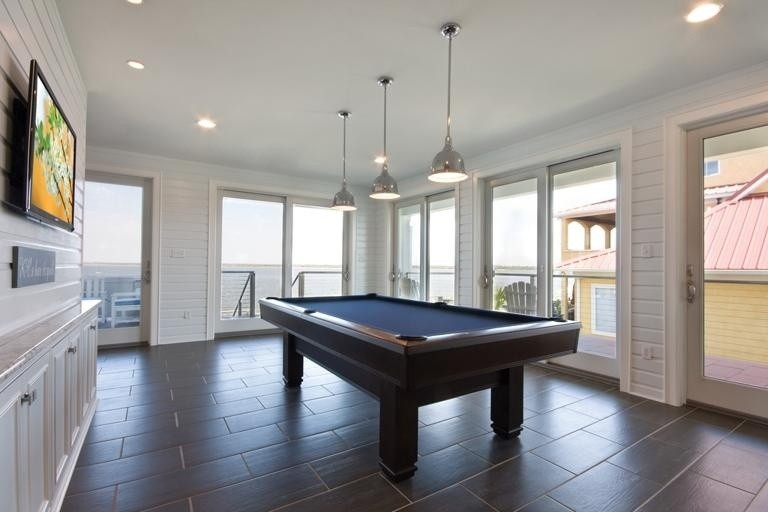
[82,271,141,328]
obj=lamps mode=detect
[329,22,468,211]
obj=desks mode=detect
[259,293,582,484]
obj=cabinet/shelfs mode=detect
[0,309,98,512]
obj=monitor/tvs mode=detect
[23,59,78,233]
